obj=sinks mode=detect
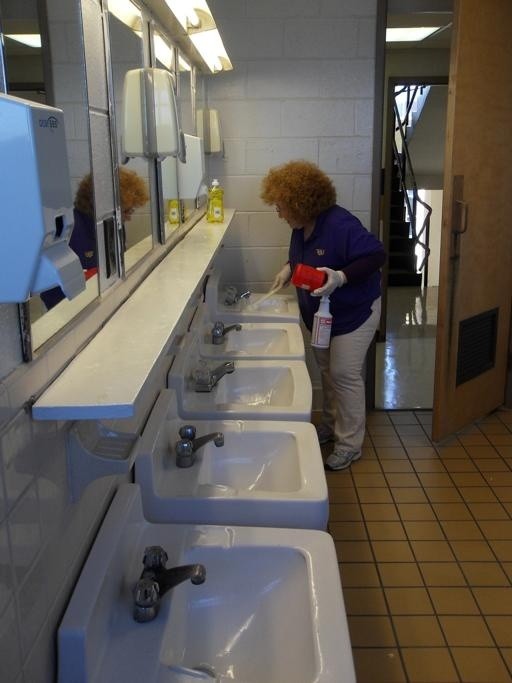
[135,388,330,530]
[53,484,356,683]
[205,269,302,324]
[188,303,306,360]
[165,334,314,422]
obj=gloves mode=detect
[270,261,293,293]
[309,266,347,298]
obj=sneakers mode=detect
[324,446,362,471]
[307,424,336,444]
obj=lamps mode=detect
[178,50,192,72]
[166,0,233,74]
[105,1,144,39]
[153,31,172,70]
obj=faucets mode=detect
[135,546,205,621]
[224,286,251,304]
[210,322,242,344]
[192,360,234,392]
[174,426,224,467]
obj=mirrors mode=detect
[1,1,104,364]
[147,20,182,244]
[174,45,201,224]
[101,1,156,272]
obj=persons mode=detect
[258,157,386,474]
[40,165,153,311]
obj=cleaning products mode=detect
[310,294,333,350]
